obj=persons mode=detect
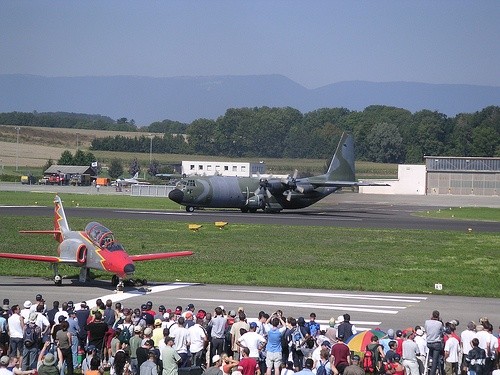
[84,357,104,375]
[444,327,462,375]
[161,336,183,375]
[188,318,208,366]
[109,351,132,375]
[343,356,366,375]
[36,340,63,375]
[263,313,287,375]
[169,317,191,367]
[331,334,350,375]
[82,345,103,375]
[425,310,444,375]
[0,294,500,375]
[401,331,421,375]
[56,321,75,375]
[465,338,486,375]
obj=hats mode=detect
[388,329,394,339]
[338,315,344,322]
[250,322,259,327]
[402,330,407,335]
[420,326,425,331]
[321,341,331,348]
[407,330,413,338]
[218,306,224,310]
[297,317,305,323]
[393,354,400,360]
[440,326,451,333]
[329,317,335,326]
[352,355,360,360]
[416,329,423,335]
[0,298,236,366]
[238,307,244,312]
[310,313,316,317]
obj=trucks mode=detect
[21,170,110,187]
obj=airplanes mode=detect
[0,192,194,295]
[156,132,391,213]
[111,172,151,192]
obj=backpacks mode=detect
[23,324,37,344]
[122,325,132,343]
[225,324,232,341]
[316,360,329,375]
[363,344,380,373]
[291,326,304,345]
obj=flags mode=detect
[92,162,97,166]
